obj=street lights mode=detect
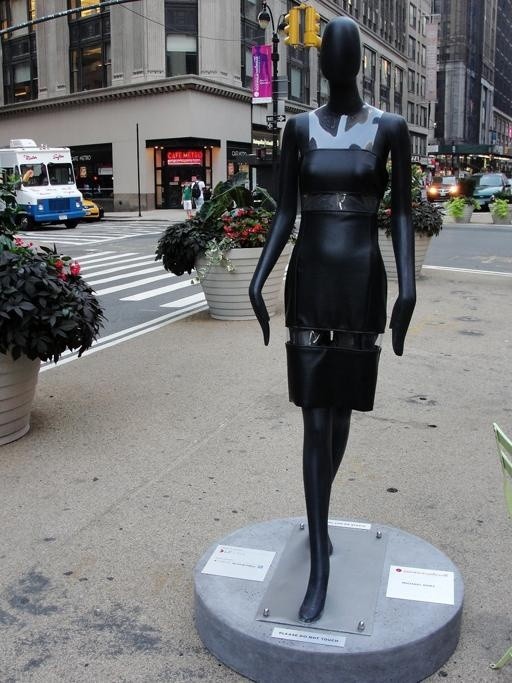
[258,1,290,179]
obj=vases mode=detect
[0,341,42,446]
[194,242,296,322]
[377,226,434,281]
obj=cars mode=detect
[79,199,100,222]
[427,175,461,203]
[464,171,511,212]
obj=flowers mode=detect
[375,188,447,238]
[1,165,107,363]
[154,169,301,284]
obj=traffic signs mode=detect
[266,115,286,122]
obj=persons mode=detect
[248,16,416,624]
[23,166,34,185]
[181,182,193,219]
[191,176,205,213]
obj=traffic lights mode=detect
[303,5,323,50]
[284,5,301,48]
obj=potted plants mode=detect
[445,193,482,223]
[487,178,511,225]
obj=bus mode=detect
[0,137,88,230]
